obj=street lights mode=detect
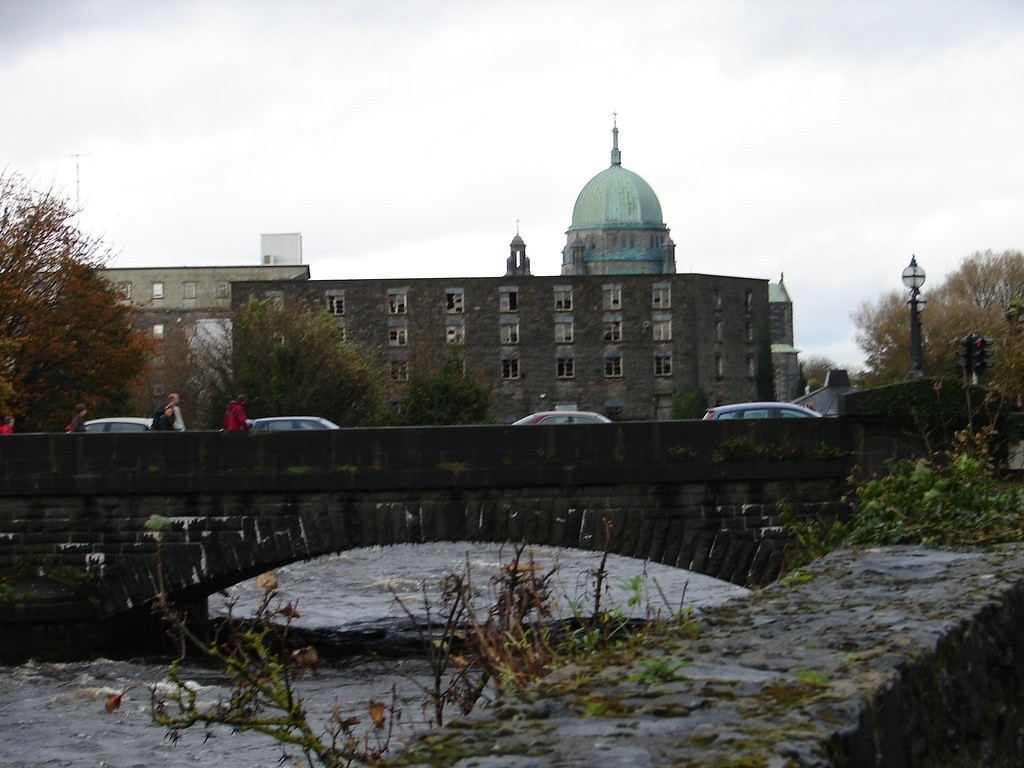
[902,254,927,373]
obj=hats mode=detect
[237,394,247,401]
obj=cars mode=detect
[219,416,340,432]
[510,411,611,425]
[697,402,823,422]
[77,416,156,435]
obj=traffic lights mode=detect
[958,330,997,375]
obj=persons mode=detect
[223,395,249,431]
[158,394,185,431]
[70,404,91,432]
[0,416,14,435]
